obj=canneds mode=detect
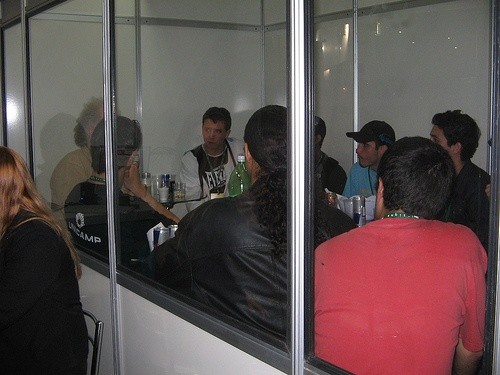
[324,193,337,208]
[352,196,367,227]
[154,228,169,249]
[168,224,178,238]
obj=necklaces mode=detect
[368,166,374,196]
[384,212,421,218]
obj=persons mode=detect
[157,107,236,203]
[314,115,347,195]
[49,96,134,212]
[0,146,89,375]
[127,105,359,336]
[64,116,180,271]
[430,109,491,257]
[342,120,396,199]
[314,137,488,375]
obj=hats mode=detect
[346,120,396,149]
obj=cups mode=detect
[354,196,366,227]
[172,183,185,200]
[142,173,175,199]
[158,187,169,203]
[343,199,353,220]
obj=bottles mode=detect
[228,155,251,197]
